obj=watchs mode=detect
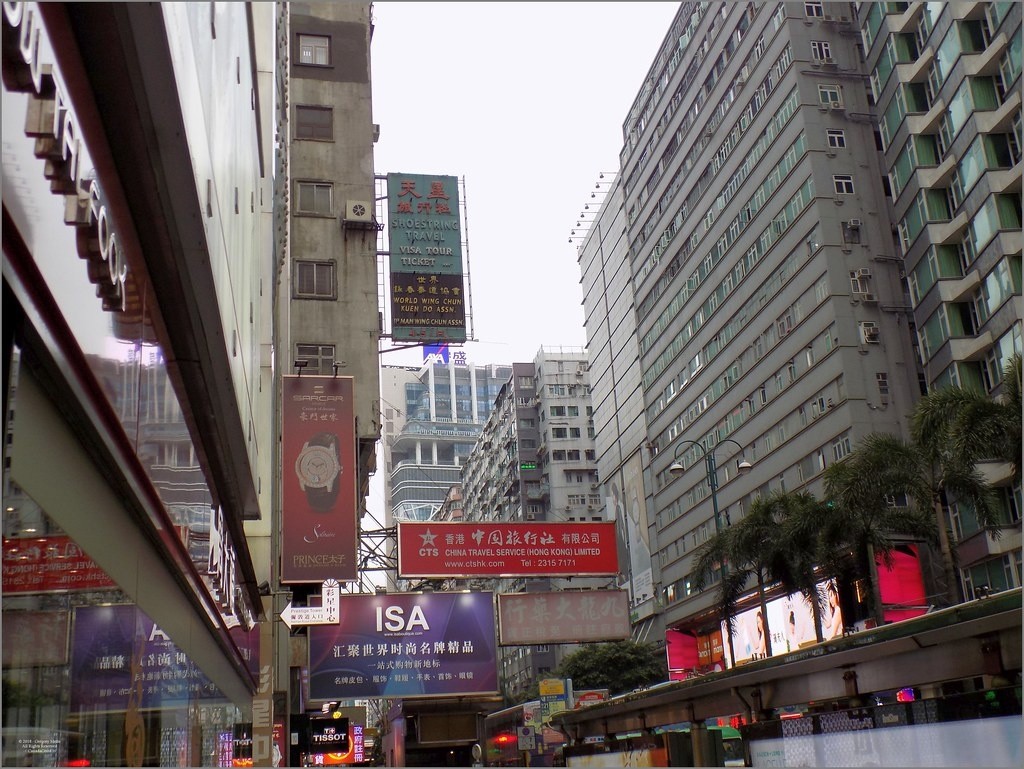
[272,741,282,767]
[295,432,343,512]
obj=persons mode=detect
[746,583,842,657]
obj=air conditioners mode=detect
[577,370,583,375]
[568,449,572,453]
[595,471,598,476]
[829,101,845,113]
[373,123,380,142]
[344,200,373,221]
[867,327,881,336]
[838,15,849,25]
[704,128,712,138]
[862,293,878,303]
[848,219,861,229]
[590,483,597,489]
[823,57,837,66]
[824,14,835,21]
[527,514,535,520]
[570,384,574,388]
[586,448,591,452]
[565,505,571,511]
[645,442,652,449]
[587,504,592,511]
[857,268,870,279]
[736,76,744,86]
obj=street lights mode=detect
[669,439,754,666]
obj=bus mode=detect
[480,701,570,768]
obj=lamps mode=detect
[636,598,641,605]
[422,586,435,595]
[257,581,271,597]
[642,594,647,601]
[331,360,349,376]
[294,359,308,376]
[629,601,633,607]
[470,582,483,593]
[374,585,387,597]
